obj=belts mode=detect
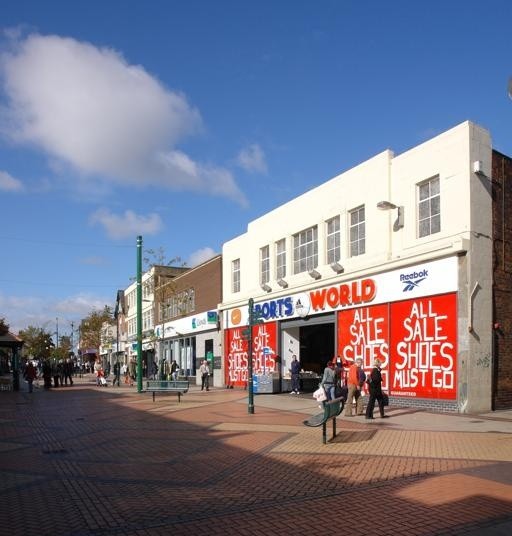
[324,382,336,385]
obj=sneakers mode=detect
[289,390,300,395]
[344,412,390,419]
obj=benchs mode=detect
[137,380,190,402]
[302,395,346,445]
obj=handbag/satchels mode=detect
[312,386,327,401]
[335,384,348,399]
[381,391,389,406]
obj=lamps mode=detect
[260,283,272,292]
[276,278,289,288]
[307,269,322,280]
[376,199,405,227]
[330,261,344,273]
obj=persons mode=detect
[1,357,180,395]
[318,354,367,417]
[199,360,211,391]
[365,359,389,419]
[288,355,302,395]
